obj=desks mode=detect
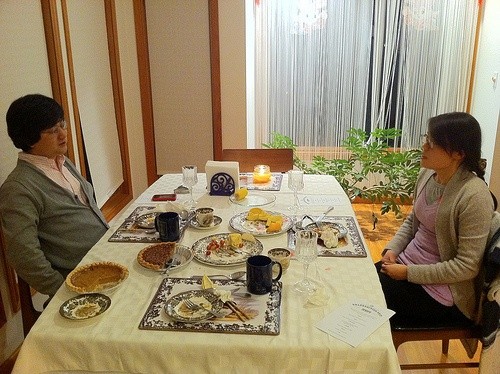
[12,173,402,374]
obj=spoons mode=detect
[178,210,188,228]
[192,271,246,280]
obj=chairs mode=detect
[388,212,500,372]
[219,148,293,173]
[15,275,52,340]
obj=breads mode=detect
[247,207,283,232]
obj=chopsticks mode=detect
[226,301,250,323]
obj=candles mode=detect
[254,164,272,184]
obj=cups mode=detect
[194,207,214,227]
[268,248,291,274]
[246,255,282,295]
[155,212,180,242]
[253,166,270,182]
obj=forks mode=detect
[183,299,223,318]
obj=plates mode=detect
[134,212,165,229]
[229,210,294,235]
[59,292,112,321]
[65,262,129,296]
[191,233,264,266]
[164,290,224,322]
[190,215,222,230]
[137,243,195,272]
[229,190,276,211]
[303,221,348,240]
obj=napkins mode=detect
[206,160,241,191]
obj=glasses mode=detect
[421,134,450,153]
[42,120,67,135]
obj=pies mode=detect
[227,233,242,248]
[137,242,176,270]
[65,261,128,292]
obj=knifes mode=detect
[315,205,334,222]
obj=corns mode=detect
[235,187,248,201]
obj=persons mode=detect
[375,111,498,327]
[0,94,110,300]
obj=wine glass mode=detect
[182,165,199,207]
[286,169,304,212]
[294,231,319,295]
